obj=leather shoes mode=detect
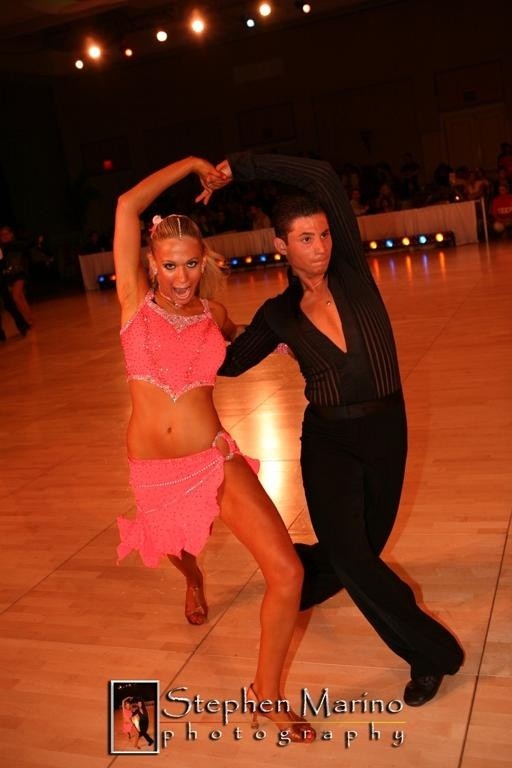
[404,639,465,707]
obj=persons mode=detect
[1,225,36,343]
[194,153,466,707]
[87,159,512,256]
[113,155,316,743]
[121,697,154,748]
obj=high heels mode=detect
[184,568,208,625]
[243,684,315,743]
